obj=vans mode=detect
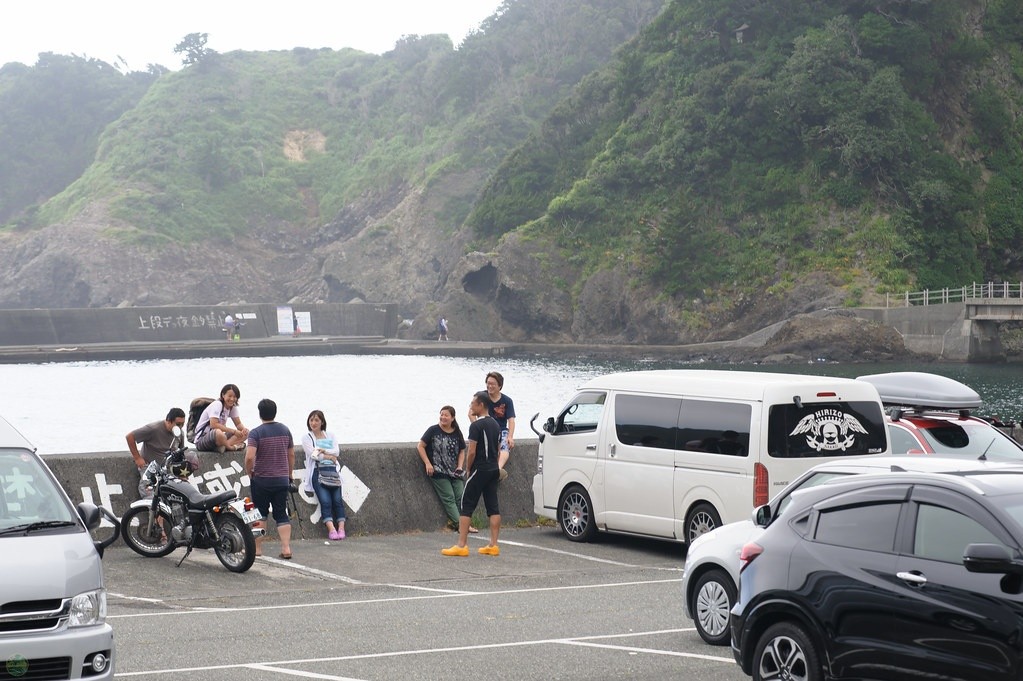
[0,414,123,681]
[532,368,890,550]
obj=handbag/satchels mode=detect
[318,470,341,487]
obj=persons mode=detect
[194,384,249,453]
[685,430,746,457]
[245,398,295,558]
[417,406,478,532]
[293,315,298,336]
[633,435,658,447]
[468,372,516,481]
[438,315,449,341]
[126,408,185,546]
[302,410,345,540]
[441,391,501,556]
[225,311,241,341]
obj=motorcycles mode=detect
[119,426,266,573]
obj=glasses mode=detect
[173,420,184,427]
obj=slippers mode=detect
[243,549,261,555]
[279,552,293,559]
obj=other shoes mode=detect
[233,443,246,451]
[214,446,225,453]
[159,535,167,546]
[468,525,478,532]
[498,469,508,483]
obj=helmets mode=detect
[169,460,196,479]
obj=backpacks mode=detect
[186,397,224,443]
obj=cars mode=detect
[730,464,1023,681]
[854,371,1022,461]
[683,454,1023,647]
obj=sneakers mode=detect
[338,528,345,539]
[441,545,469,556]
[477,545,500,555]
[328,529,339,540]
